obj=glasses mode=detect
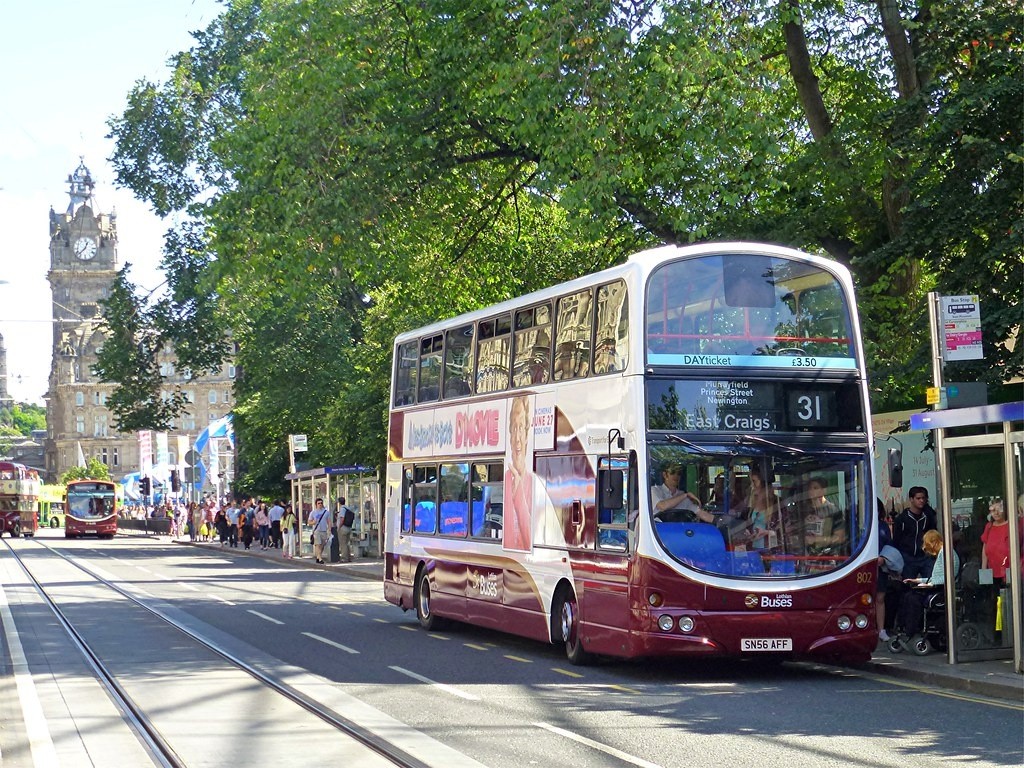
[666,468,682,475]
[317,502,323,504]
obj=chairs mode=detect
[648,333,807,356]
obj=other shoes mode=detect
[316,558,319,563]
[190,539,282,551]
[287,555,293,560]
[878,629,890,641]
[282,552,286,557]
[318,560,323,564]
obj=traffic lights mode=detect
[139,477,150,495]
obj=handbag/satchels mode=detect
[995,596,1003,630]
[309,534,314,544]
[184,524,189,533]
[283,527,287,533]
[212,527,217,538]
[200,523,209,536]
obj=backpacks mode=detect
[342,505,355,528]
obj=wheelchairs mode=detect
[886,560,980,655]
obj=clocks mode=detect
[73,237,98,260]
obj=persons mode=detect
[118,503,155,521]
[853,484,961,642]
[503,396,532,552]
[335,496,352,564]
[711,468,846,574]
[280,505,299,559]
[26,467,41,481]
[979,493,1024,648]
[308,498,331,565]
[649,464,715,523]
[214,496,303,551]
[150,498,221,544]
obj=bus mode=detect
[0,460,41,539]
[62,480,120,540]
[37,482,125,528]
[384,242,905,667]
